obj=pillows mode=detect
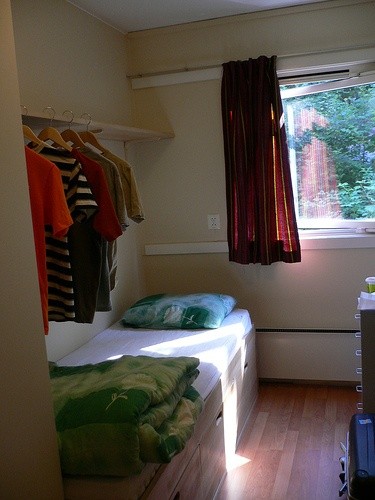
[123,291,238,330]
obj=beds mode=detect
[49,307,259,499]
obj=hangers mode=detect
[21,104,112,155]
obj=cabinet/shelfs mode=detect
[356,291,375,414]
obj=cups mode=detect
[365,276,375,294]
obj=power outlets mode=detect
[208,215,220,229]
